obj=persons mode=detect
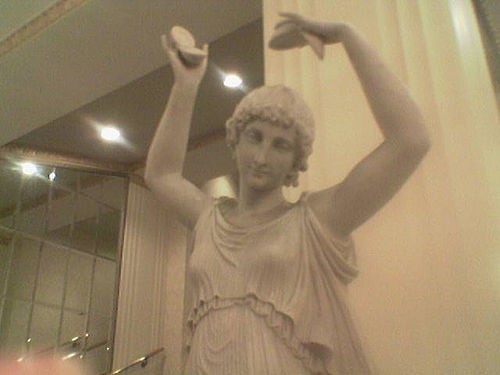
[144,10,431,374]
